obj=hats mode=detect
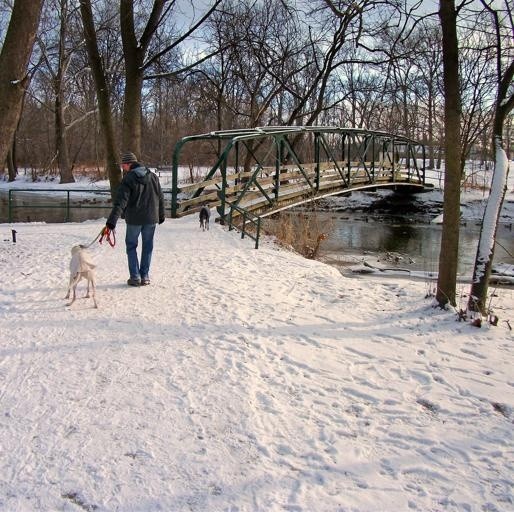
[120,151,137,164]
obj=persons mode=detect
[102,150,166,289]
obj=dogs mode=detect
[199,204,211,232]
[64,245,98,308]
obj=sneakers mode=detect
[127,279,150,287]
